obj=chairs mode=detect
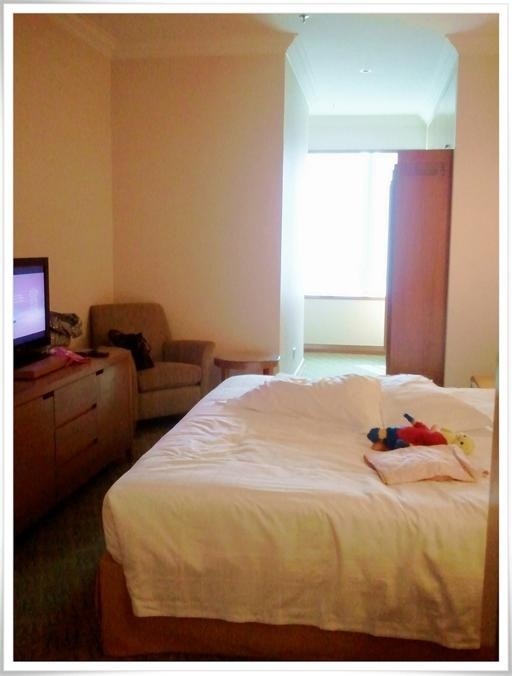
[87,301,216,422]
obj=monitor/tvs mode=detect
[13,257,51,369]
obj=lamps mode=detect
[296,14,312,25]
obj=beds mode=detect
[97,372,499,660]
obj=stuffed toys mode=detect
[365,411,479,457]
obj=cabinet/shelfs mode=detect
[13,345,133,537]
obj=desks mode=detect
[469,374,496,388]
[213,348,280,385]
